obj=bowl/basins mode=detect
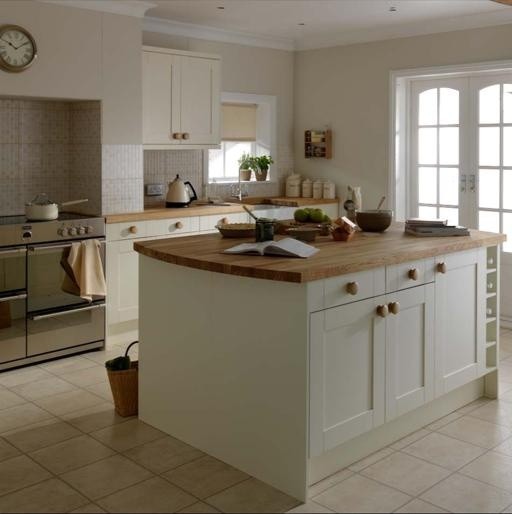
[284,224,355,242]
[215,224,255,237]
[354,209,393,231]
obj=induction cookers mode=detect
[0,211,106,247]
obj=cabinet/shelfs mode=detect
[303,126,332,160]
[200,211,253,235]
[106,214,200,349]
[433,240,503,422]
[141,44,222,151]
[299,256,435,489]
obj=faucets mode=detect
[238,160,263,200]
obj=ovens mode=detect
[0,238,105,372]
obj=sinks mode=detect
[249,204,291,224]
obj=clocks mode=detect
[1,23,38,73]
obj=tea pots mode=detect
[164,173,197,208]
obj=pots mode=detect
[23,193,88,220]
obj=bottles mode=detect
[306,125,328,157]
[286,172,335,199]
[255,217,274,243]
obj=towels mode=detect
[66,238,107,305]
[60,245,81,295]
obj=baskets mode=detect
[104,339,149,422]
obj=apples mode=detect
[294,208,329,223]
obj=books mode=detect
[223,237,320,259]
[405,217,470,237]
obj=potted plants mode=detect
[250,154,275,181]
[237,152,259,180]
